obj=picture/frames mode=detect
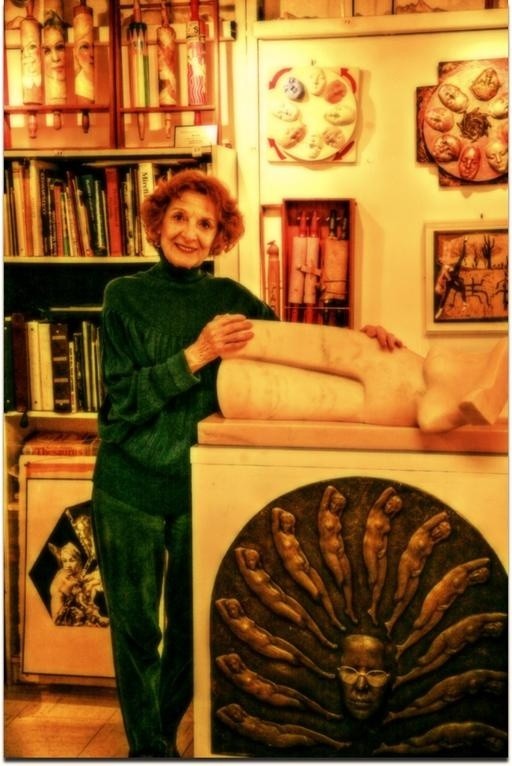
[425,217,510,334]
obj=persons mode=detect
[46,542,110,628]
[268,66,357,160]
[38,11,69,107]
[212,485,507,756]
[424,68,507,181]
[89,169,405,759]
[18,16,45,106]
[72,13,96,100]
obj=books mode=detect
[3,302,105,413]
[4,157,207,257]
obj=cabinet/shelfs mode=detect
[4,144,243,692]
